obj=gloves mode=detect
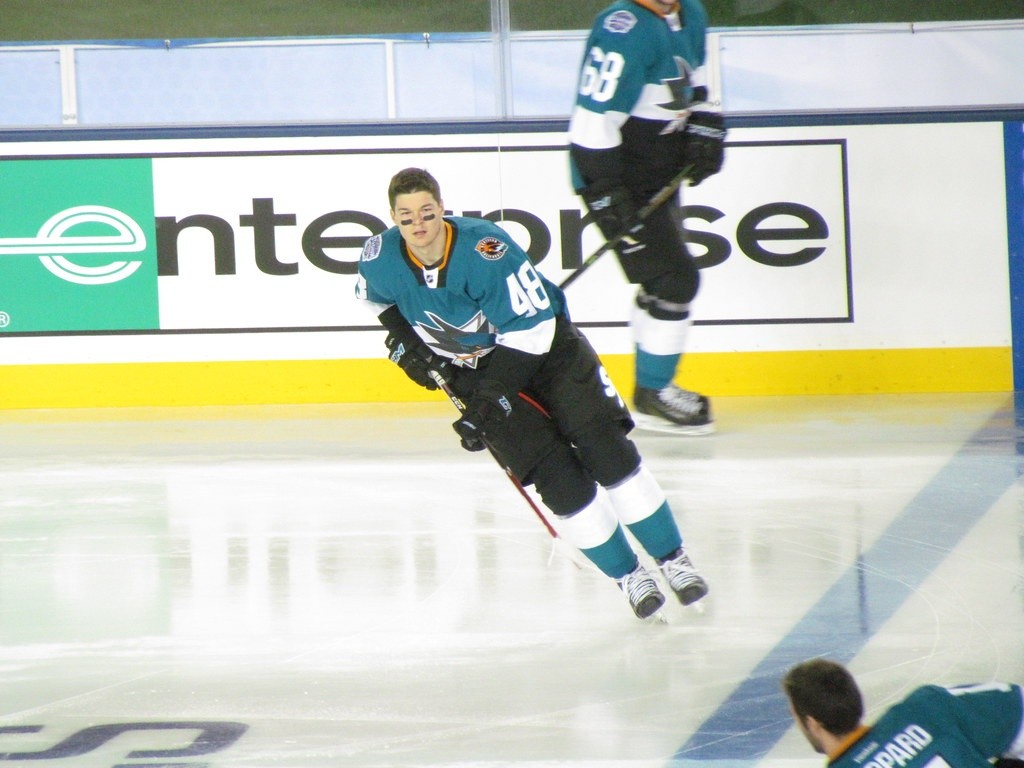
[452,379,513,452]
[385,334,454,391]
[677,111,727,187]
[582,178,649,256]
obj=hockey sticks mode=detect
[558,162,695,290]
[427,369,586,573]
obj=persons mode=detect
[354,167,711,626]
[569,0,718,435]
[783,659,1024,768]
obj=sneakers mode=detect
[630,382,715,434]
[655,547,710,612]
[616,555,668,627]
